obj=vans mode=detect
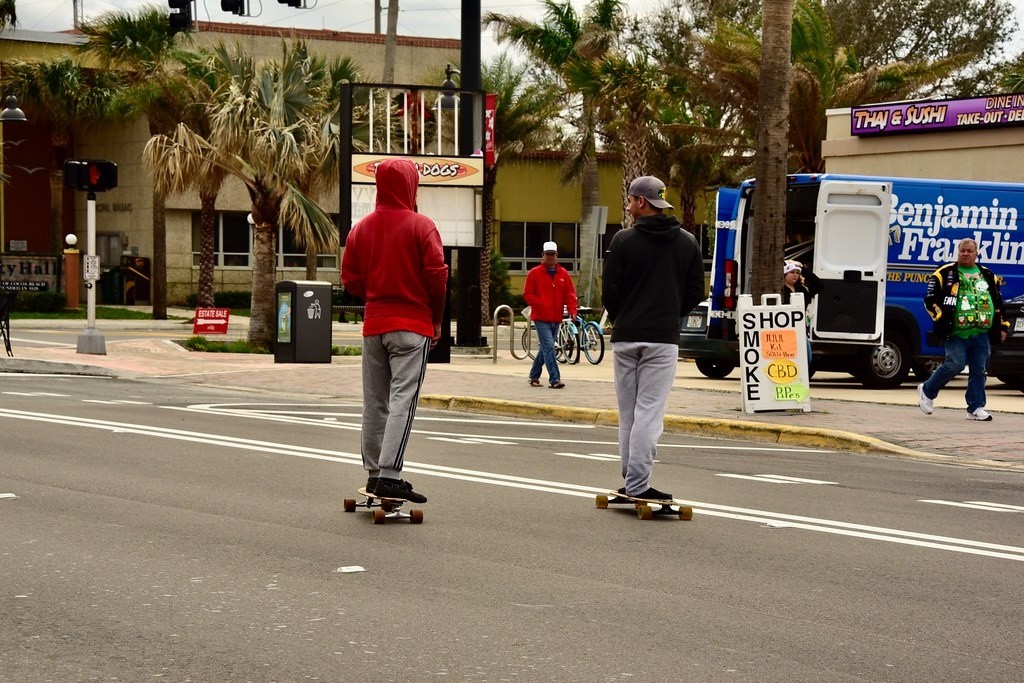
[705,172,1024,393]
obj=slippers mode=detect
[528,380,543,387]
[548,382,565,388]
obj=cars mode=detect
[676,300,742,379]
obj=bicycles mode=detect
[521,295,606,366]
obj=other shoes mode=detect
[618,487,626,494]
[634,488,673,500]
[373,479,428,503]
[365,477,379,493]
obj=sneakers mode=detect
[917,384,933,414]
[967,408,992,421]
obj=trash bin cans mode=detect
[274,280,333,363]
[102,269,123,304]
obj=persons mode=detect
[341,159,448,503]
[918,237,1010,421]
[523,241,578,389]
[601,176,704,500]
[782,258,823,365]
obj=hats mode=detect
[543,242,558,254]
[628,174,675,210]
[783,260,803,275]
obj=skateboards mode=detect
[595,489,694,521]
[341,486,424,525]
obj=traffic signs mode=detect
[193,308,230,334]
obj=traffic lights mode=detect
[278,0,302,8]
[168,0,194,30]
[65,160,120,192]
[220,0,244,16]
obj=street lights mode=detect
[430,63,488,351]
